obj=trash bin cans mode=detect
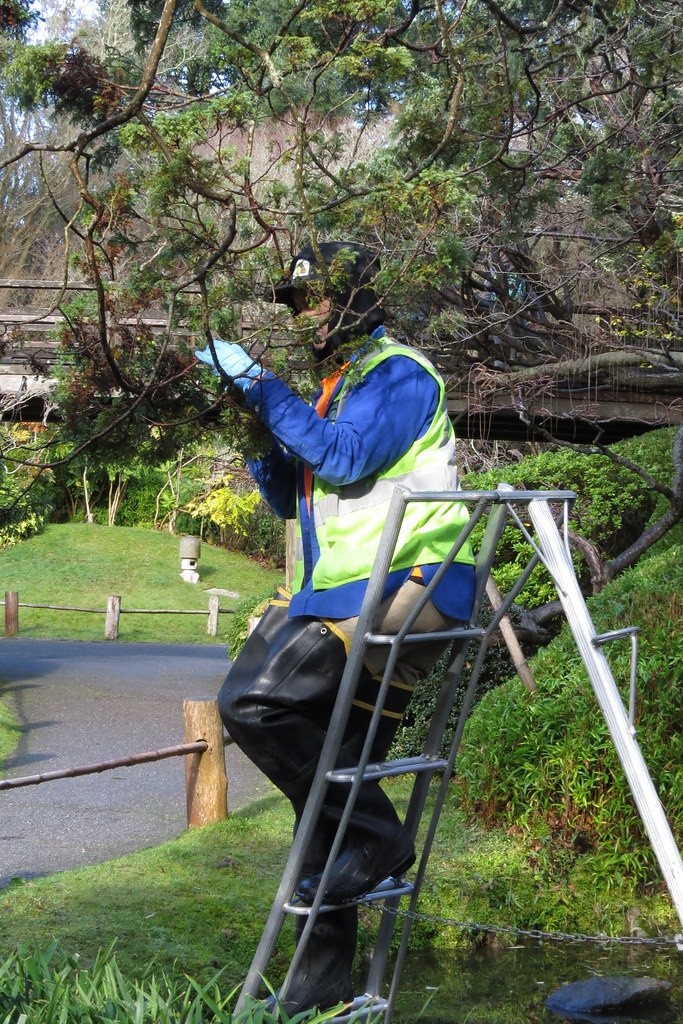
[179,535,201,584]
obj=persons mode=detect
[193,237,474,1024]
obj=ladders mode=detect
[232,482,682,1024]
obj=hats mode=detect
[263,241,381,303]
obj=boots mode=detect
[217,601,417,908]
[245,674,417,1022]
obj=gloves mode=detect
[195,339,266,390]
